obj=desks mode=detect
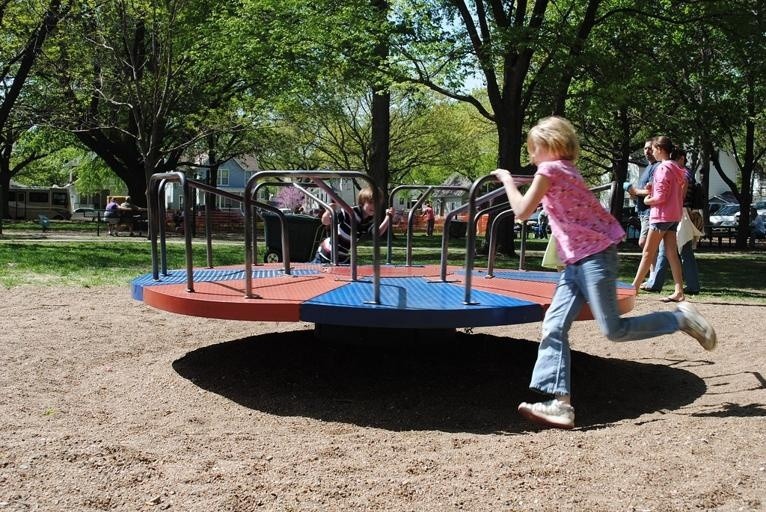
[705,224,735,245]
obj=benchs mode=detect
[87,209,263,236]
[705,232,737,246]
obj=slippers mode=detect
[660,294,684,303]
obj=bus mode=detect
[8,188,73,220]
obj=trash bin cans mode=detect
[448,220,467,237]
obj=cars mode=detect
[73,207,105,218]
[709,200,766,235]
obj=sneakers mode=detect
[675,300,715,351]
[517,395,575,429]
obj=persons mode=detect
[628,136,701,302]
[104,195,147,237]
[490,115,716,430]
[421,203,435,237]
[748,208,766,248]
[537,208,551,236]
[307,186,396,264]
